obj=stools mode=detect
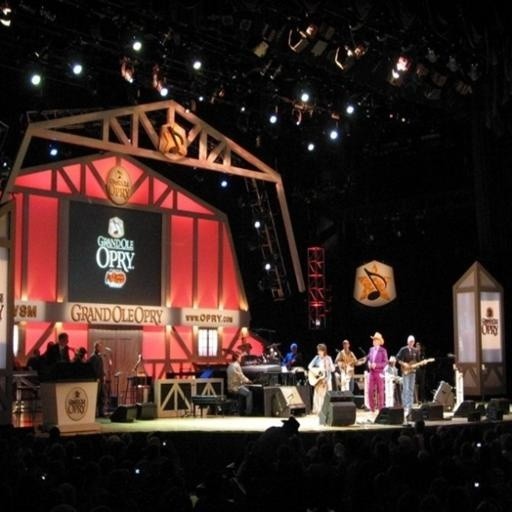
[227,392,247,416]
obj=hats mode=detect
[282,417,299,435]
[370,332,384,344]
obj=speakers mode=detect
[486,399,509,416]
[136,403,157,420]
[468,412,480,421]
[375,407,404,424]
[419,402,444,420]
[320,391,355,426]
[109,404,138,422]
[487,408,503,420]
[453,400,474,418]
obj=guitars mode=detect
[401,357,435,375]
[338,356,367,375]
[307,363,339,387]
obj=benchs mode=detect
[189,394,240,419]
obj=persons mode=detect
[24,333,112,417]
[283,332,425,417]
[226,351,253,415]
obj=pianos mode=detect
[218,364,282,417]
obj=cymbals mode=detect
[237,343,252,351]
[267,343,281,349]
[222,348,237,356]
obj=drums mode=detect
[278,371,287,386]
[296,371,304,386]
[133,373,152,390]
[287,371,297,386]
